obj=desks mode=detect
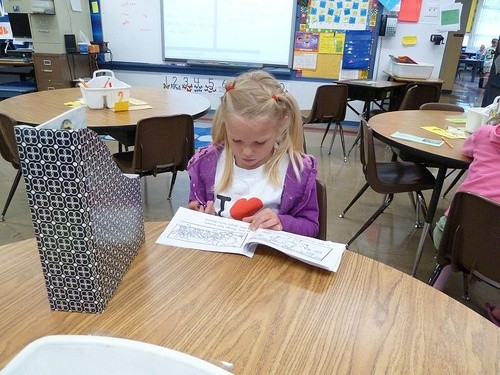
[0,87,212,136]
[0,61,36,82]
[0,221,500,375]
[384,69,445,110]
[459,60,484,83]
[368,110,474,278]
[66,52,110,87]
[333,79,406,155]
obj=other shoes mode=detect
[485,303,500,327]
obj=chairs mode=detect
[110,126,136,153]
[113,113,195,201]
[298,83,499,289]
[0,113,22,221]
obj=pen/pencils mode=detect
[103,78,112,87]
[442,137,453,148]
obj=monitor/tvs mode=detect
[8,13,33,42]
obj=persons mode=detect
[482,38,498,77]
[186,72,320,238]
[476,44,486,59]
[433,124,500,327]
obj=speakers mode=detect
[64,34,76,53]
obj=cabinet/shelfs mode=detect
[3,0,97,91]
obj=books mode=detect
[157,206,348,274]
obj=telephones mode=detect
[378,14,397,38]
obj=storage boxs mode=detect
[13,124,145,315]
[78,70,131,109]
[391,60,434,79]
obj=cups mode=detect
[78,43,88,54]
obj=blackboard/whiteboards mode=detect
[89,0,297,79]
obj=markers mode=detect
[171,64,186,67]
[79,78,89,88]
